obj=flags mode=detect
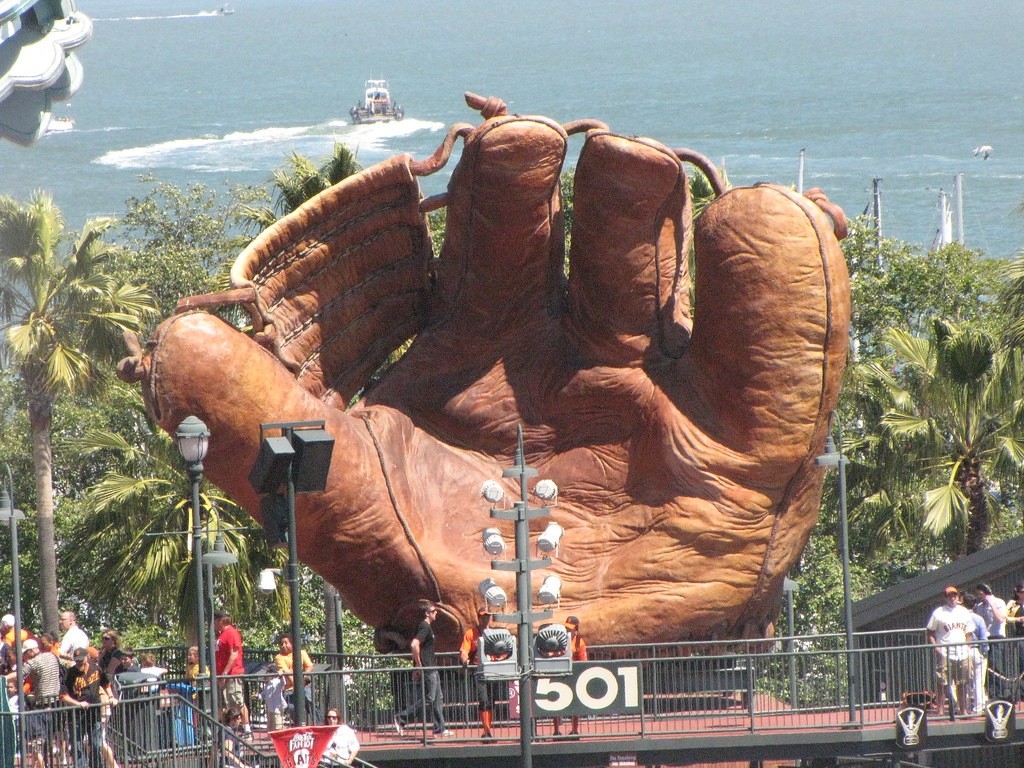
[269,727,339,768]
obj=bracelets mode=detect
[109,695,115,699]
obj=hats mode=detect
[943,586,958,598]
[563,616,579,631]
[207,609,228,620]
[21,639,38,653]
[74,648,87,661]
[477,606,491,616]
[1,614,15,629]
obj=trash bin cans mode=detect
[166,681,196,748]
[115,671,160,755]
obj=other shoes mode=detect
[394,714,404,736]
[238,731,253,746]
[480,733,497,744]
[552,731,579,741]
[432,729,454,738]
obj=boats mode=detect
[217,3,235,15]
[350,79,404,123]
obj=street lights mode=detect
[0,462,28,768]
[480,480,573,768]
[502,423,540,739]
[176,415,212,742]
[203,507,239,741]
[815,409,857,722]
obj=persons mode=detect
[461,607,509,744]
[214,610,252,734]
[0,611,141,768]
[319,710,359,768]
[217,710,248,768]
[142,651,168,708]
[925,584,1024,715]
[550,616,587,741]
[251,667,292,739]
[187,647,211,727]
[274,636,323,725]
[393,600,458,738]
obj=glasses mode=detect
[101,637,111,641]
[326,716,338,719]
[429,608,436,613]
